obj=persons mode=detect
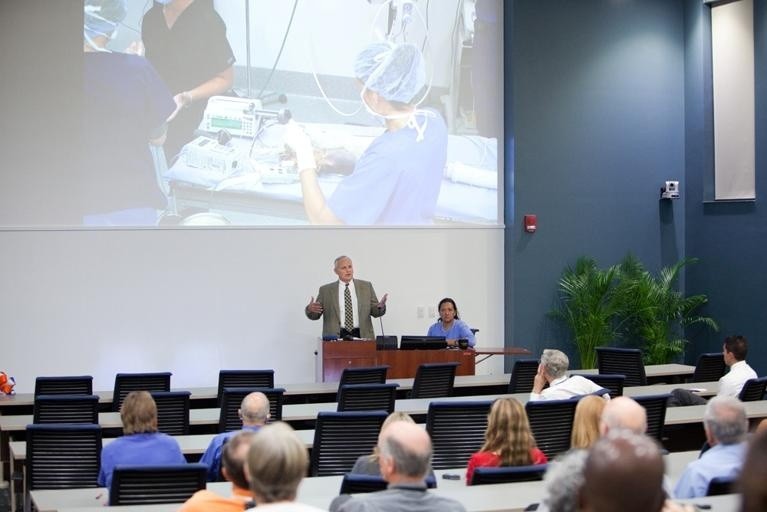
[287,43,448,227]
[427,298,476,348]
[305,256,388,339]
[142,0,237,170]
[81,0,177,227]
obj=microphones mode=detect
[377,305,384,341]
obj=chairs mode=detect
[0,336,767,509]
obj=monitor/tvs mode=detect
[401,335,448,349]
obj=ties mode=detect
[344,283,353,333]
